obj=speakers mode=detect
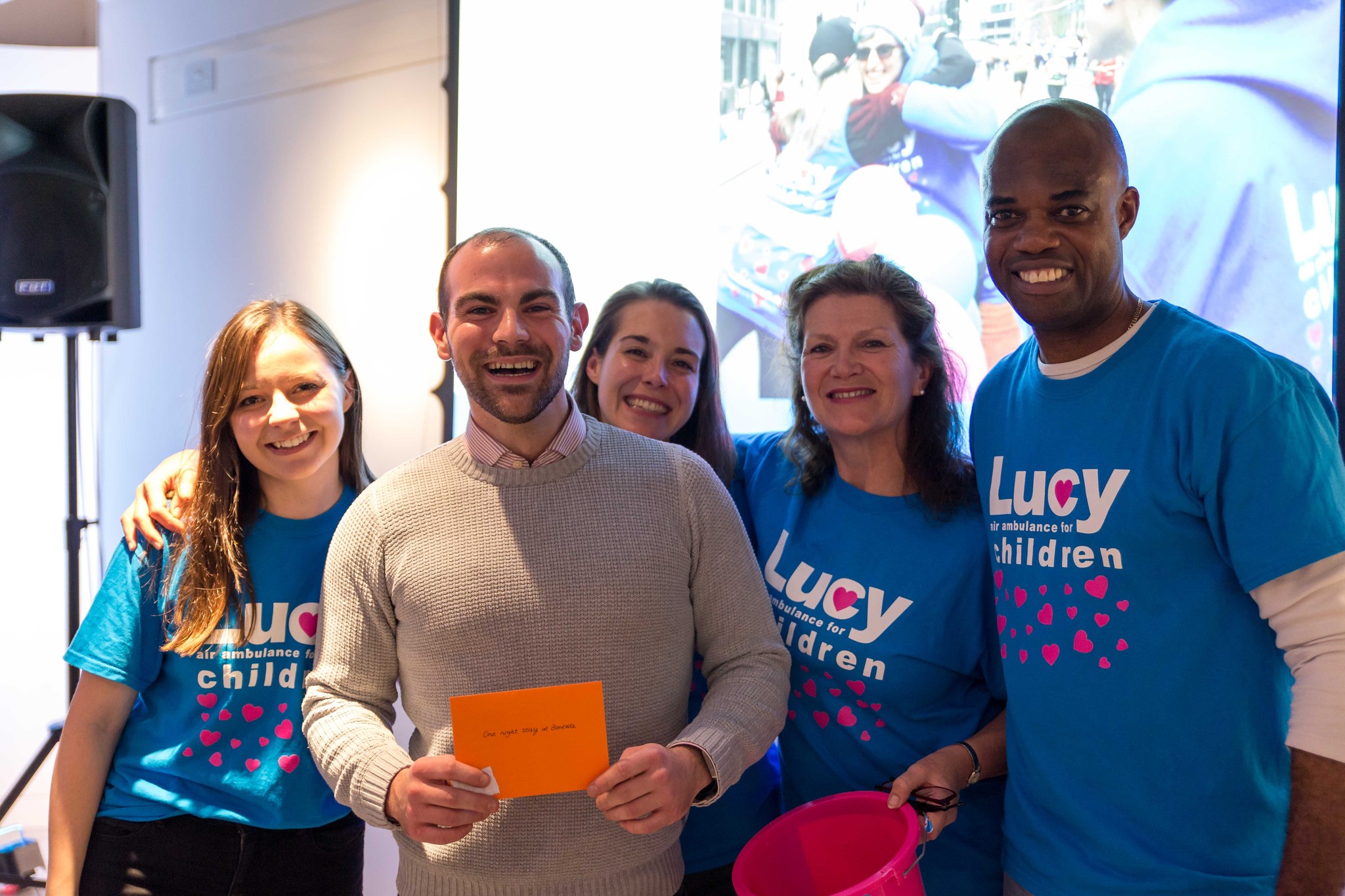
[0,91,140,341]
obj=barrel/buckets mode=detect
[731,791,930,896]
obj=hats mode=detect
[808,14,854,76]
[853,2,938,83]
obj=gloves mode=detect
[850,81,904,146]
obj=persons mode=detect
[121,226,792,895]
[966,100,1345,896]
[709,0,1340,399]
[299,227,791,895]
[40,296,378,895]
[731,252,1010,895]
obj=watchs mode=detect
[959,741,980,788]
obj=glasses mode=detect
[857,44,902,63]
